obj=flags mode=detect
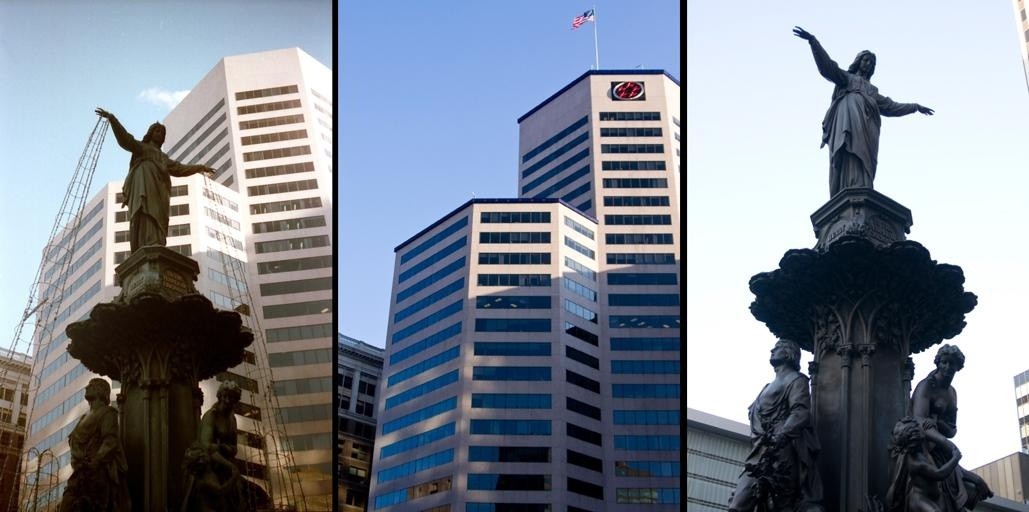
[570,8,594,30]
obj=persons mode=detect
[179,440,241,512]
[93,106,218,254]
[197,379,245,512]
[911,343,966,511]
[728,338,813,512]
[59,377,130,512]
[793,25,935,188]
[884,415,963,512]
